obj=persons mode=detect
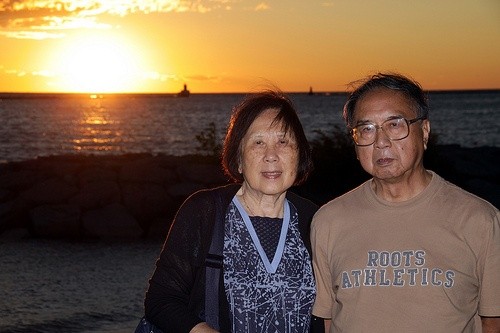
[144,89,324,333]
[310,72,500,333]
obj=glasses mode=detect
[349,116,423,147]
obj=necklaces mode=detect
[242,185,254,216]
